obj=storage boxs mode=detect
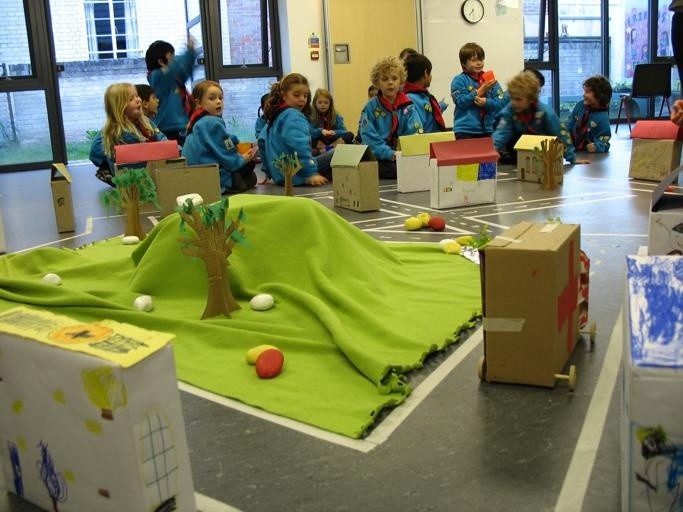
[477,220,581,389]
[1,306,197,512]
[648,166,682,257]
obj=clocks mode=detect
[461,0,484,23]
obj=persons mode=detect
[672,100,682,126]
[451,43,505,139]
[355,48,449,180]
[88,40,258,193]
[630,28,639,70]
[641,44,648,64]
[565,74,613,153]
[492,68,589,165]
[256,73,354,186]
[659,31,669,56]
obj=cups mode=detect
[481,69,496,86]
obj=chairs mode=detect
[615,63,672,135]
